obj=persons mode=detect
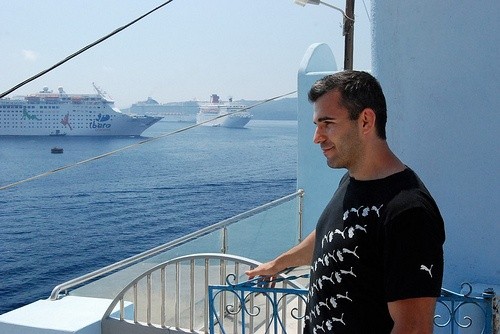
[245,69,446,334]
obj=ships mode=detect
[195,102,251,128]
[0,85,162,140]
[141,110,196,123]
